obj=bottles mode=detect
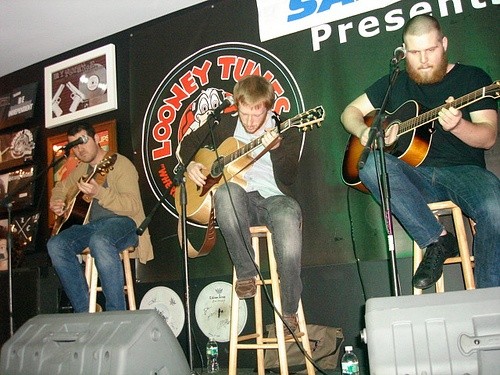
[205,336,219,374]
[340,346,360,375]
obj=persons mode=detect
[340,14,500,290]
[46,121,154,313]
[175,75,303,337]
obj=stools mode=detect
[412,200,476,295]
[229,225,316,375]
[82,246,136,313]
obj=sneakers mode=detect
[283,312,299,336]
[234,278,257,299]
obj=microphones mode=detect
[392,47,407,64]
[64,134,89,152]
[207,95,235,120]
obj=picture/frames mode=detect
[47,120,117,228]
[44,43,117,129]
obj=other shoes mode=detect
[96,303,102,312]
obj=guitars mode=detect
[174,104,326,226]
[52,153,118,237]
[339,79,500,194]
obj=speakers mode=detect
[0,309,191,375]
[359,287,500,375]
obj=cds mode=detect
[79,64,107,100]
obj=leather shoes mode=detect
[413,231,458,289]
